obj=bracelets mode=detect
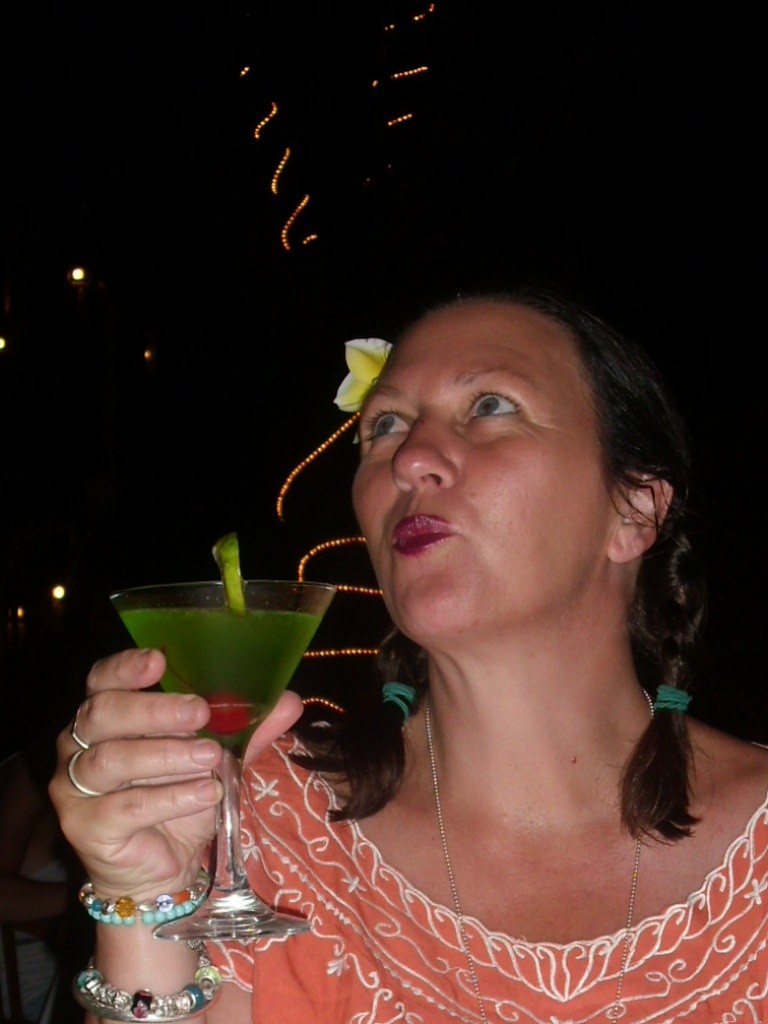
[69,937,222,1021]
[79,869,218,925]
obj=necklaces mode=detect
[423,688,657,1024]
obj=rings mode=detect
[69,702,94,753]
[68,750,107,796]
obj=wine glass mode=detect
[109,579,335,941]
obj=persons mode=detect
[1,745,86,1024]
[49,279,768,1024]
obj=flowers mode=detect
[332,336,392,444]
